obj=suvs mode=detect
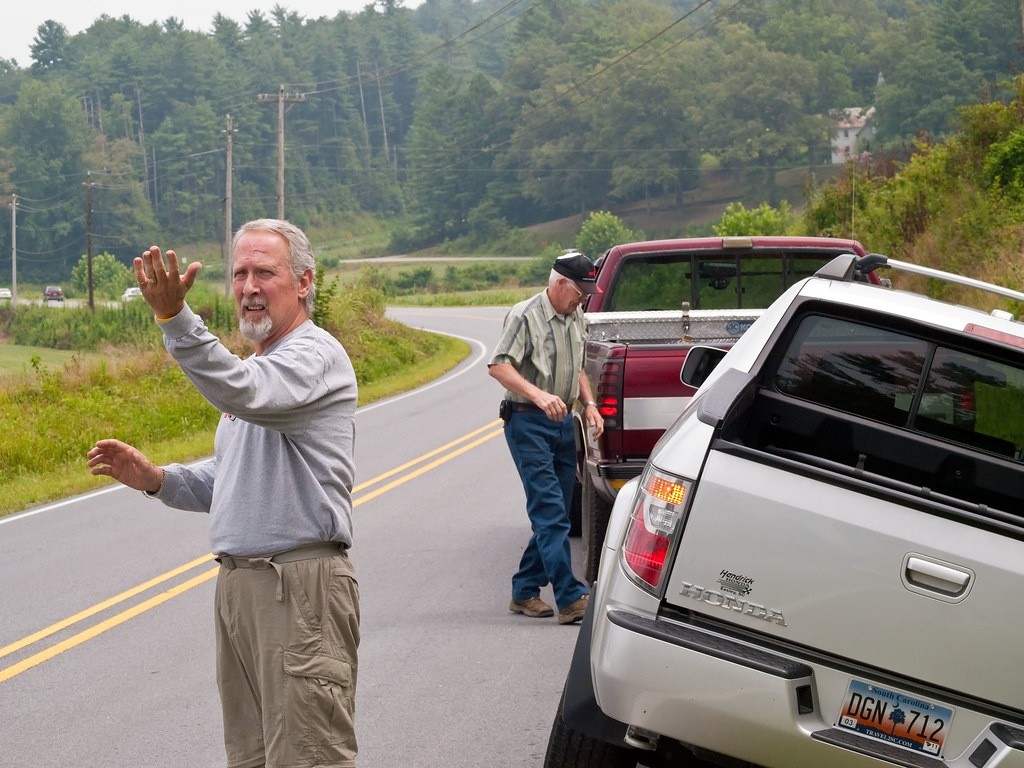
[542,251,1024,768]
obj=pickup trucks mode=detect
[569,236,977,588]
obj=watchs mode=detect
[585,401,597,407]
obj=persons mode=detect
[487,252,606,625]
[87,217,363,768]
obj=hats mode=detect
[552,252,604,294]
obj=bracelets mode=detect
[143,466,165,500]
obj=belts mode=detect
[516,403,572,413]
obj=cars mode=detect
[0,288,12,300]
[121,288,145,302]
[43,286,64,302]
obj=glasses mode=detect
[566,280,591,300]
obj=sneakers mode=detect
[558,594,589,624]
[509,597,554,617]
